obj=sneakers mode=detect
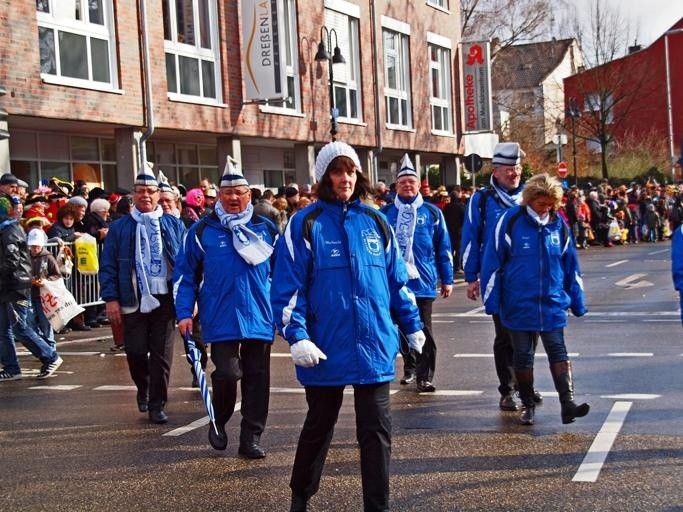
[37,355,64,379]
[1,368,23,381]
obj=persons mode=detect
[456,142,543,412]
[268,141,428,512]
[170,154,281,460]
[2,157,683,385]
[98,160,188,424]
[479,172,590,425]
[376,151,455,392]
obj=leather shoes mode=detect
[60,318,109,334]
[137,393,148,412]
[416,380,435,392]
[499,394,522,411]
[208,421,228,450]
[400,374,416,384]
[238,439,266,458]
[149,409,169,424]
[532,390,543,402]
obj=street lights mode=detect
[554,116,562,162]
[661,26,682,179]
[564,94,582,187]
[313,24,347,143]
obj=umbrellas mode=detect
[178,324,219,437]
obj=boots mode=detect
[549,360,589,424]
[513,366,535,425]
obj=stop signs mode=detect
[557,161,568,178]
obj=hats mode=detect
[186,188,205,208]
[397,151,420,177]
[314,140,362,184]
[221,155,249,188]
[491,142,525,166]
[28,228,45,247]
[136,161,159,186]
[157,169,173,193]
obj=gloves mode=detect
[290,341,327,368]
[406,330,427,355]
